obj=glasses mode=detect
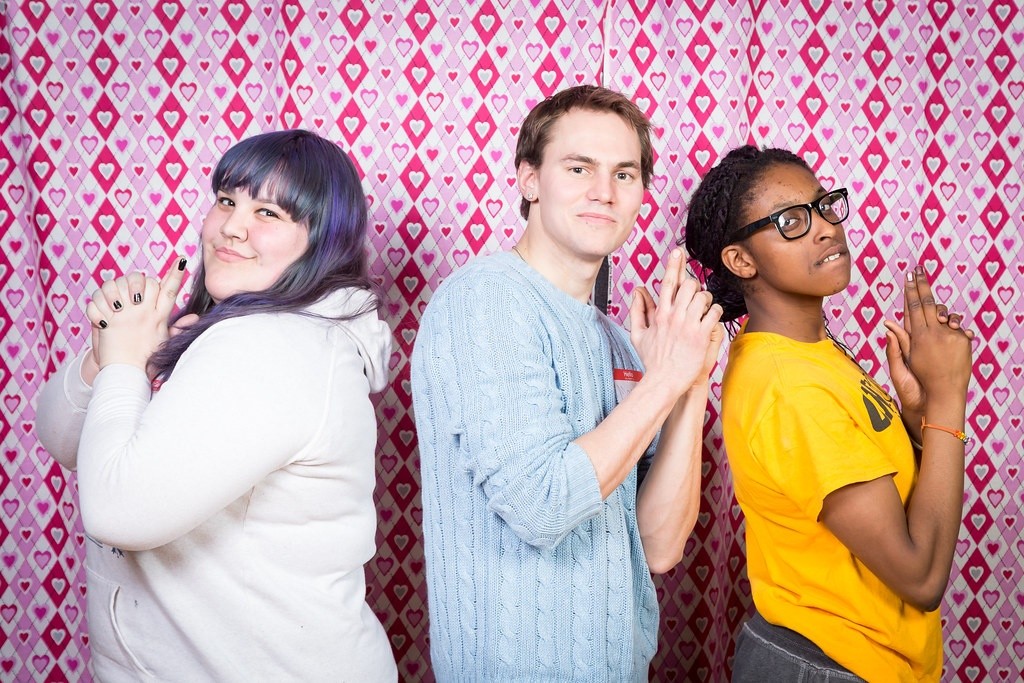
[730,188,849,241]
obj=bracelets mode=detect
[919,416,969,444]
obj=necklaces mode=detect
[511,245,527,264]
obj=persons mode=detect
[36,129,398,683]
[410,84,725,682]
[677,145,974,682]
